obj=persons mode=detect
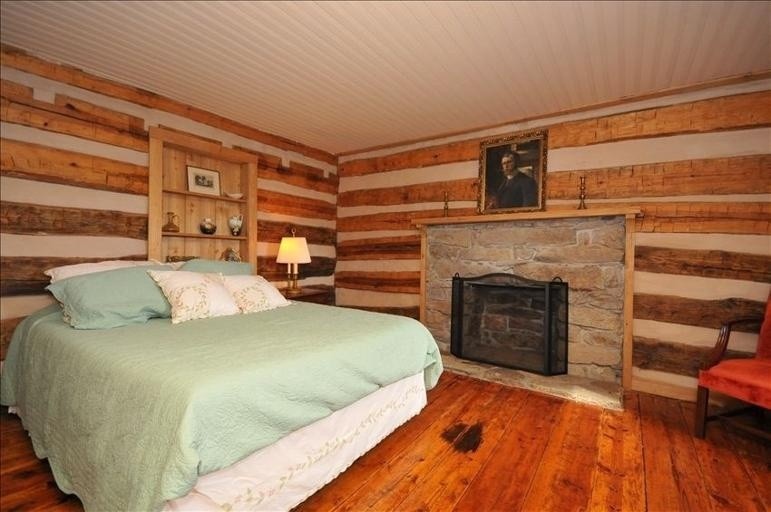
[488,152,535,207]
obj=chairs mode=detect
[692,290,771,442]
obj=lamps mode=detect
[275,229,312,293]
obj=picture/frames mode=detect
[477,127,549,216]
[186,163,222,196]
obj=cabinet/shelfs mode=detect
[147,125,256,276]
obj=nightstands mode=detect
[279,288,330,304]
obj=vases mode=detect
[199,218,218,235]
[162,212,181,233]
[228,214,245,236]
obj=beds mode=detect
[2,299,441,512]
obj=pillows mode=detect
[147,267,241,326]
[175,260,252,275]
[42,265,136,282]
[46,264,176,328]
[221,272,291,317]
